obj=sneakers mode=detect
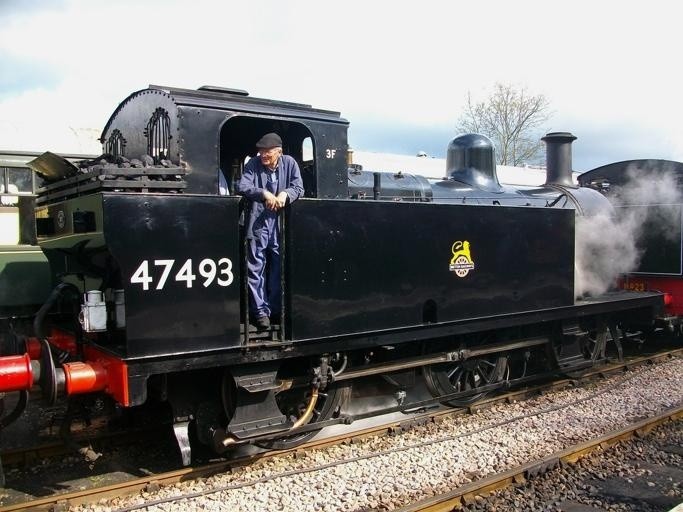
[257,316,270,328]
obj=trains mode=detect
[0,85,683,466]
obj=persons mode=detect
[238,131,304,333]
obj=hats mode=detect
[255,132,283,150]
